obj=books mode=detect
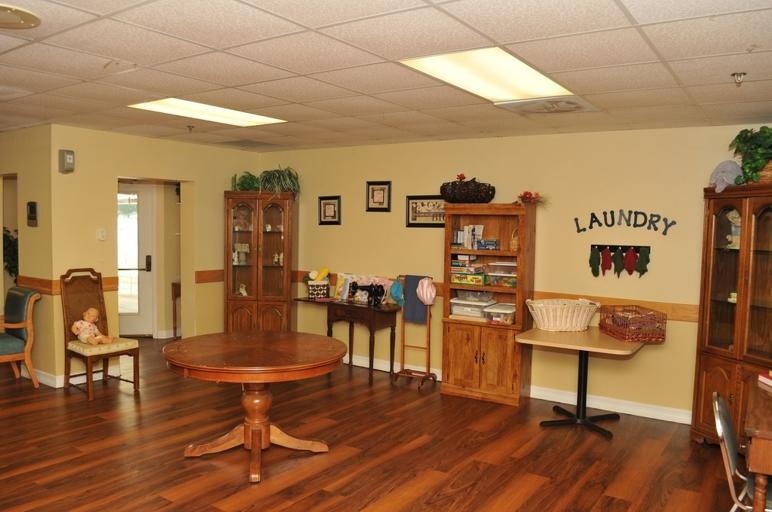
[757,370,772,387]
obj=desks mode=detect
[744,376,772,512]
[515,326,645,441]
[163,332,347,484]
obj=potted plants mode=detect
[728,125,772,184]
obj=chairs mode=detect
[712,392,772,512]
[0,287,41,389]
[60,268,139,401]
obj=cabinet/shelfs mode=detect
[689,183,772,456]
[440,201,536,407]
[223,191,299,332]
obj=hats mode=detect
[388,275,407,308]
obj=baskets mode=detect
[523,296,602,333]
[599,303,669,346]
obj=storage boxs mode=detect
[448,297,497,318]
[483,303,515,325]
[488,262,518,274]
[488,273,517,288]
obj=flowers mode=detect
[518,192,543,203]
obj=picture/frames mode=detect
[318,195,341,225]
[366,180,391,211]
[406,195,445,228]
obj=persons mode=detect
[71,307,113,345]
